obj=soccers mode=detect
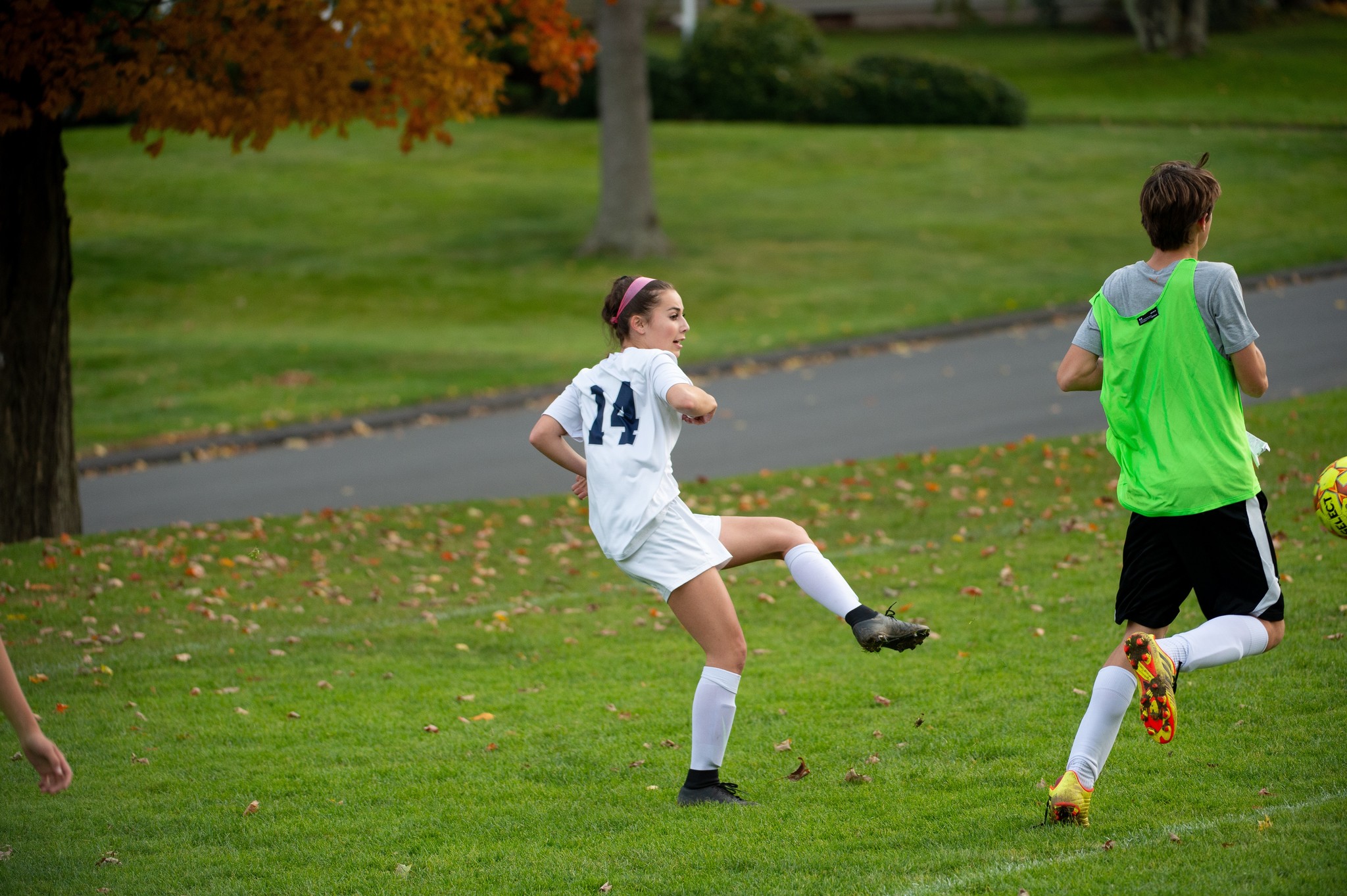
[1311,453,1347,540]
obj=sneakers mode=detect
[852,602,930,653]
[677,782,758,807]
[1124,632,1182,744]
[1031,771,1094,829]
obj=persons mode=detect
[530,275,930,807]
[1027,153,1285,827]
[0,636,73,795]
